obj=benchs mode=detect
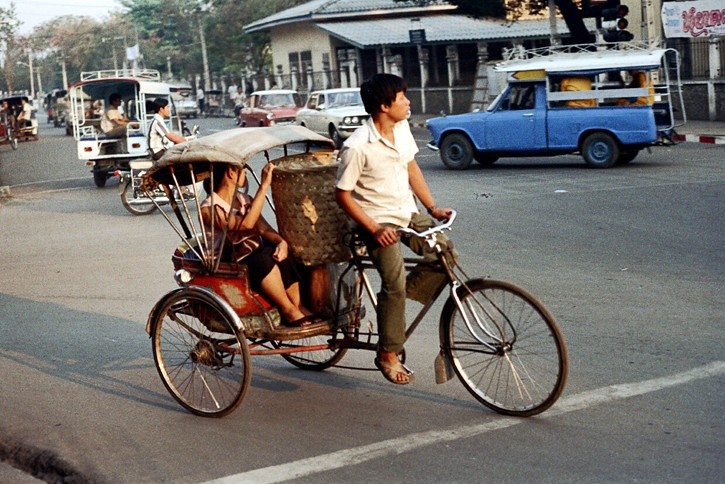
[127,134,149,153]
[80,137,124,156]
[598,101,670,117]
[173,234,295,328]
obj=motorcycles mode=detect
[69,68,194,188]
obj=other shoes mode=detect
[303,313,322,323]
[427,142,439,151]
[233,117,237,120]
[286,316,312,328]
[179,191,195,199]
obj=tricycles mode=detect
[133,124,569,419]
[0,95,39,150]
[198,89,226,118]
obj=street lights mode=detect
[15,48,34,99]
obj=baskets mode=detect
[267,152,367,266]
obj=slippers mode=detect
[374,358,409,384]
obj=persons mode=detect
[335,74,458,384]
[0,81,281,200]
[520,67,655,109]
[197,159,322,328]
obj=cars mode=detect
[238,89,304,128]
[295,87,373,150]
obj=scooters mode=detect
[120,124,249,216]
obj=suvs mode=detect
[168,83,199,118]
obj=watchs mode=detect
[428,206,438,214]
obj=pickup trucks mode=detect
[425,46,675,171]
